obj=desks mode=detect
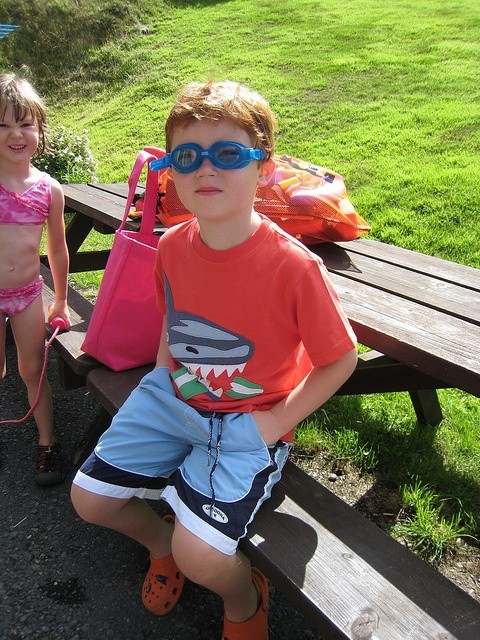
[40,183,480,428]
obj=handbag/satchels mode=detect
[80,151,163,372]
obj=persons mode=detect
[70,80,358,640]
[0,73,69,486]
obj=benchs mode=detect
[40,260,480,640]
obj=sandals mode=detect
[141,514,185,617]
[221,566,269,640]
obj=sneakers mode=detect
[35,443,67,488]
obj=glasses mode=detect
[149,140,266,174]
